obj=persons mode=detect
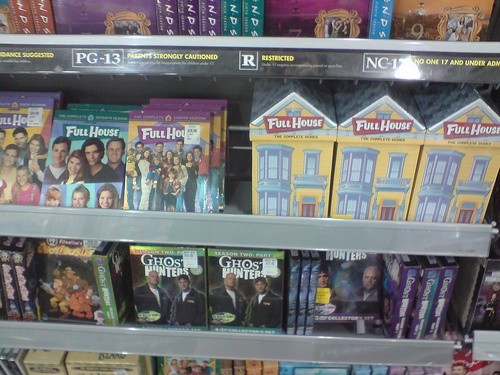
[0,126,500,375]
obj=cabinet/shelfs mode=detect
[0,35,500,367]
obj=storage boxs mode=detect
[24,349,68,375]
[66,352,146,375]
